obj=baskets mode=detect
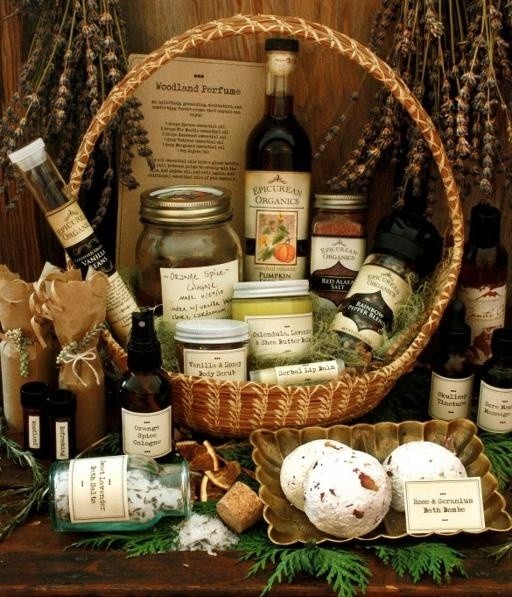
[64,15,466,439]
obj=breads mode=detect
[281,438,468,539]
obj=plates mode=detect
[246,415,511,547]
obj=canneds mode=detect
[135,183,432,381]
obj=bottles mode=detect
[311,192,370,304]
[329,211,449,352]
[427,300,475,423]
[458,203,507,364]
[236,35,317,294]
[45,454,200,535]
[246,354,351,389]
[9,135,139,336]
[473,327,512,436]
[20,380,78,463]
[118,310,176,460]
